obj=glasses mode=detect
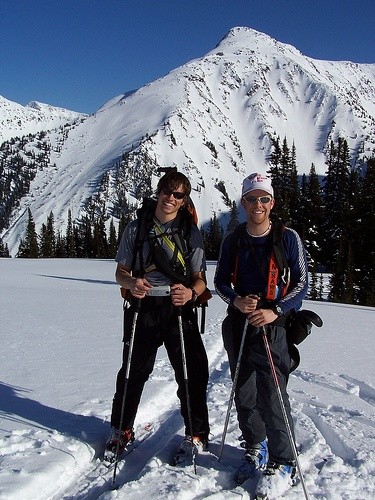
[243,196,272,204]
[160,187,186,199]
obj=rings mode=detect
[249,304,252,307]
[134,286,139,291]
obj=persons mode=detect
[213,172,309,499]
[102,170,208,463]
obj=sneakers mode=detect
[256,462,298,500]
[169,431,209,466]
[103,428,135,460]
[234,440,269,484]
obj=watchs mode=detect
[275,304,284,317]
[189,286,198,303]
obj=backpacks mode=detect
[121,197,213,313]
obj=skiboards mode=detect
[63,423,155,500]
[252,457,327,500]
[232,442,304,488]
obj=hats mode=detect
[242,173,274,198]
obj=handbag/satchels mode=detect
[285,310,323,345]
